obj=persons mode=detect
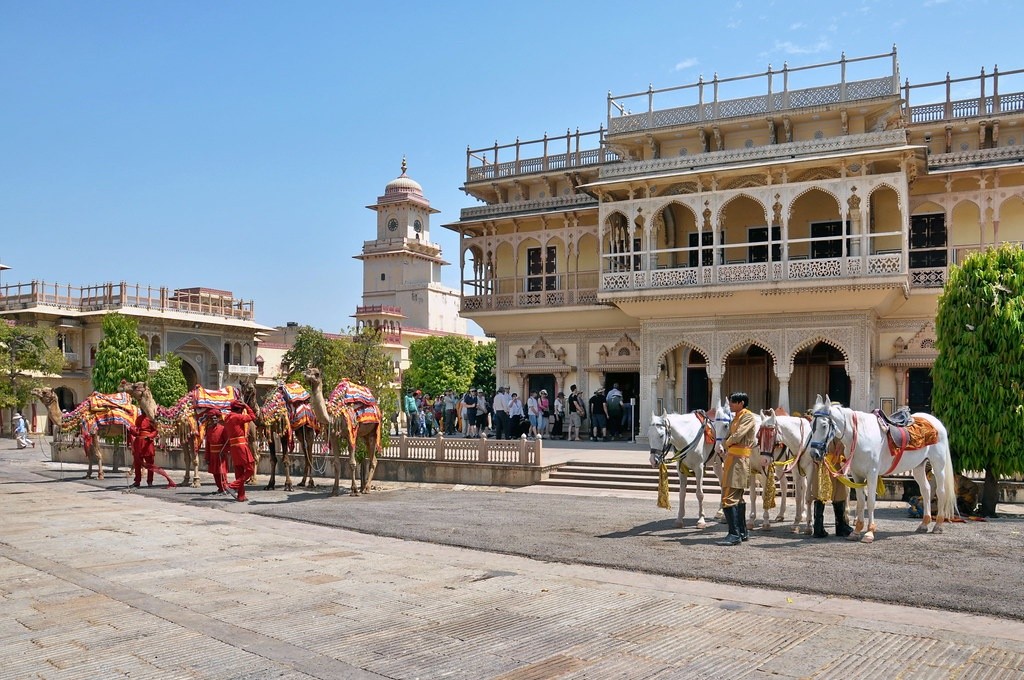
[907,460,938,519]
[224,399,256,502]
[129,407,158,487]
[204,408,228,497]
[812,436,854,538]
[952,470,978,517]
[392,382,639,442]
[13,413,35,448]
[714,392,757,546]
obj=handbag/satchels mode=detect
[542,411,550,419]
[558,411,566,422]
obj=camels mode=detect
[123,381,258,488]
[30,386,147,481]
[304,367,386,499]
[238,375,322,493]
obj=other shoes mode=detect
[477,434,481,438]
[487,434,494,438]
[129,484,140,489]
[603,438,608,441]
[594,438,597,442]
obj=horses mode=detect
[646,394,961,544]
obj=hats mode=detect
[597,388,605,392]
[208,408,222,418]
[539,389,548,396]
[477,389,483,392]
[13,413,22,419]
[230,400,245,409]
[470,389,477,391]
[406,389,414,392]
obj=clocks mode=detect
[388,218,398,231]
[414,220,422,232]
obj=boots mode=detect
[831,500,853,536]
[813,500,828,538]
[716,505,742,546]
[739,503,749,542]
